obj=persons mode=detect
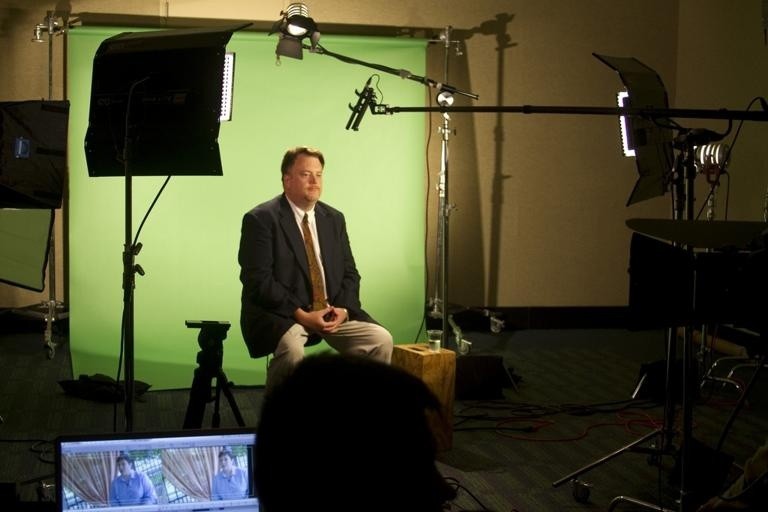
[238,147,393,391]
[211,451,249,500]
[110,456,157,506]
[253,356,455,512]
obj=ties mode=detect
[300,213,327,311]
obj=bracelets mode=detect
[342,306,351,323]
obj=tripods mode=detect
[549,205,746,487]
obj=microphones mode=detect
[346,77,371,130]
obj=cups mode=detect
[427,329,443,352]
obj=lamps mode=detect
[268,1,512,361]
[693,141,743,398]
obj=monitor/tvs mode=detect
[54,428,260,511]
